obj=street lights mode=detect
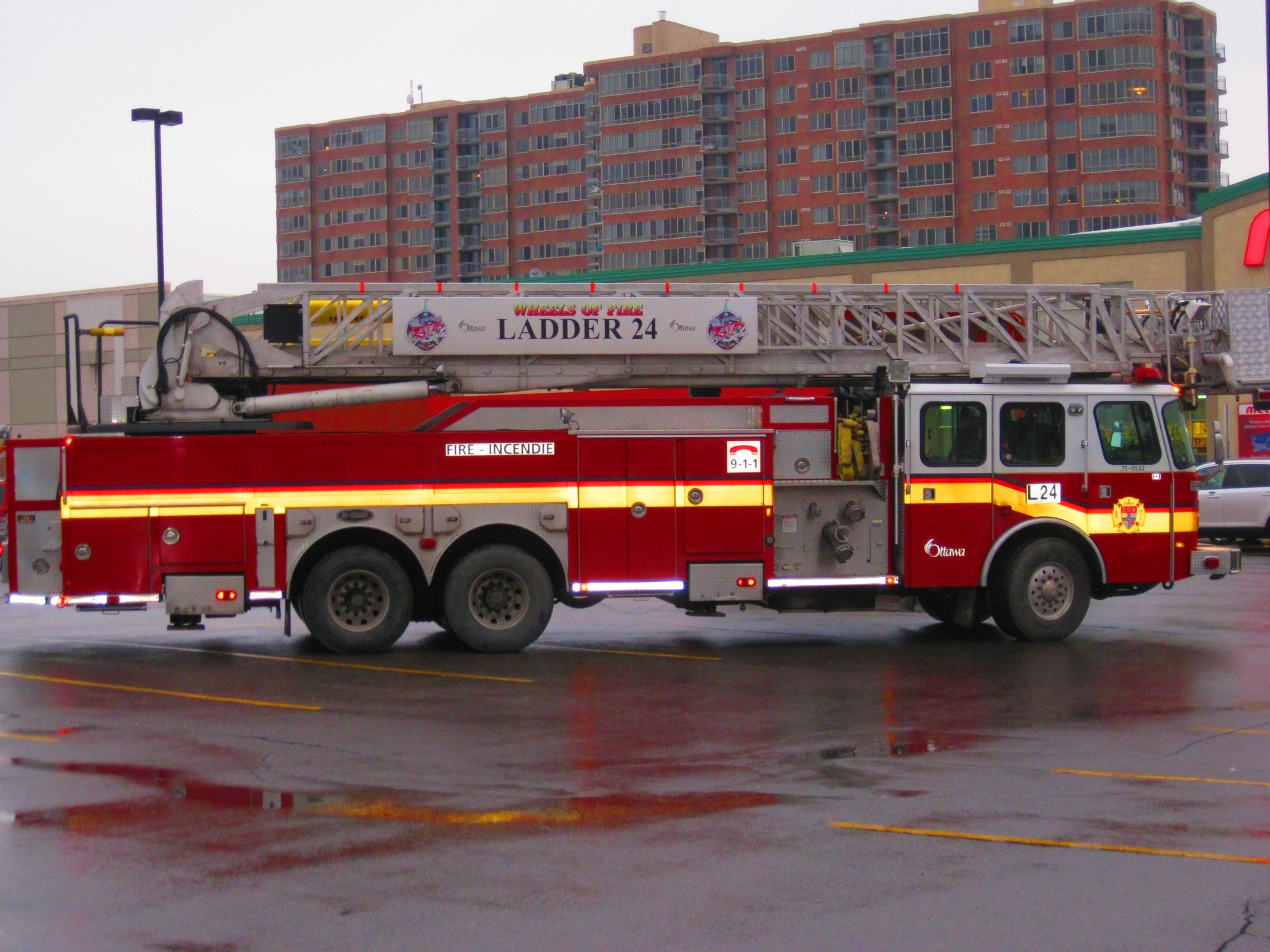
[131,108,183,303]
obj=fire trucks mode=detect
[0,278,1270,652]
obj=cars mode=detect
[1197,459,1270,543]
